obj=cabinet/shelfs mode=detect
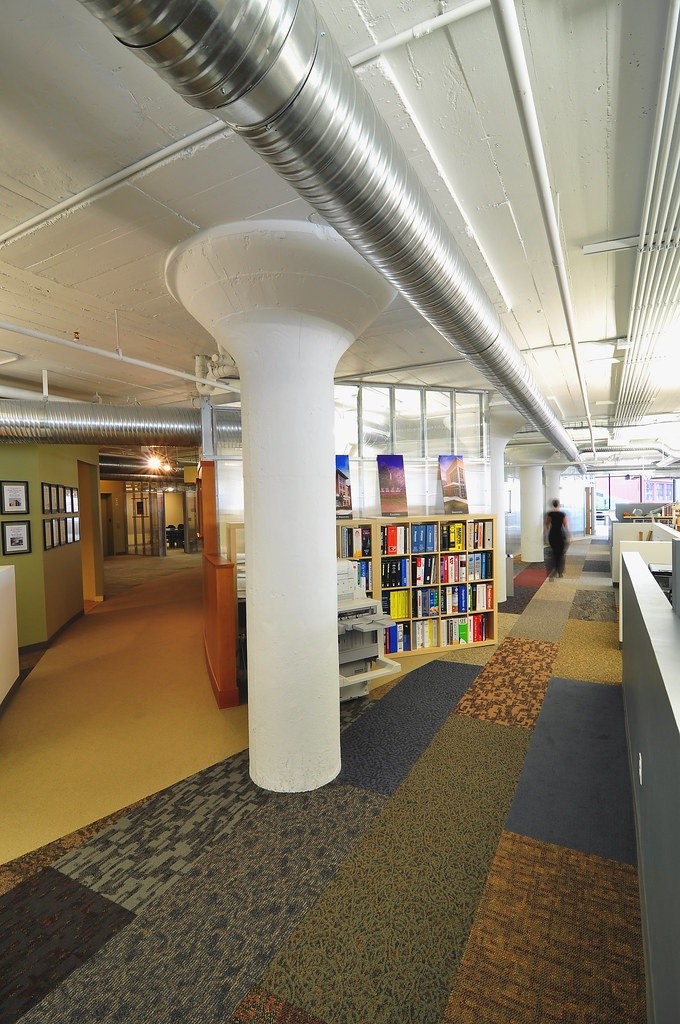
[336,513,497,660]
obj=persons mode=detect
[546,500,570,578]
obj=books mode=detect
[341,522,493,558]
[661,504,673,516]
[367,583,493,620]
[349,552,492,590]
[384,614,489,654]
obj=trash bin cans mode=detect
[183,539,197,552]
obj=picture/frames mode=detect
[1,480,80,555]
